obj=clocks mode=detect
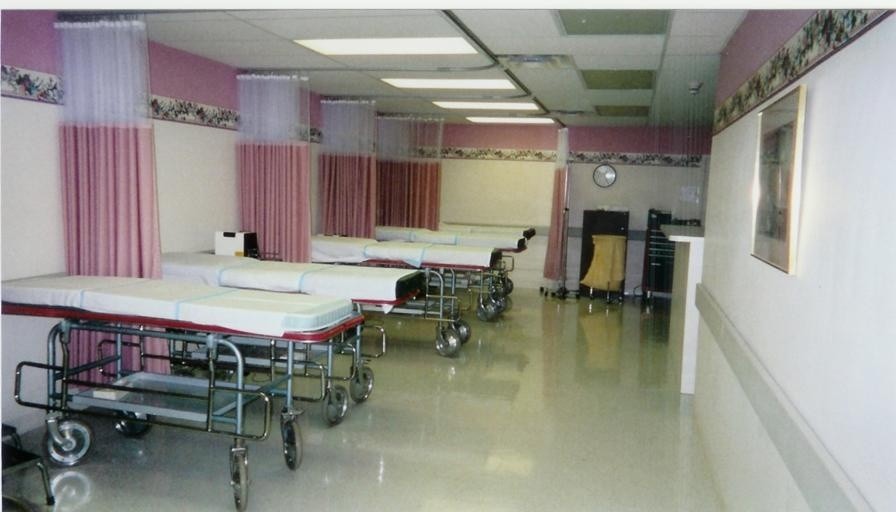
[592,164,617,188]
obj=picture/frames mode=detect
[749,83,807,276]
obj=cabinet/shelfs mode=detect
[642,226,676,314]
[580,210,629,301]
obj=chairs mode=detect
[1,424,56,510]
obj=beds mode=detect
[162,251,427,427]
[374,223,536,296]
[5,275,366,512]
[311,234,514,321]
